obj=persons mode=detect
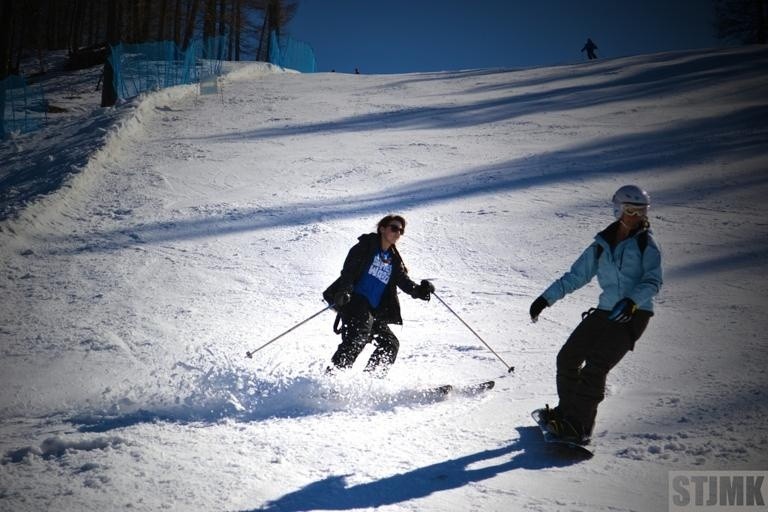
[581,38,598,60]
[322,215,435,381]
[529,185,664,445]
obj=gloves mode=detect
[530,296,547,318]
[414,280,434,300]
[608,298,637,323]
[334,289,350,307]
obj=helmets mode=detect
[613,185,649,217]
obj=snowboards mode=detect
[531,408,593,457]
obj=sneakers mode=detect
[539,409,579,439]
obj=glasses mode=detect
[387,224,404,234]
[620,204,650,216]
